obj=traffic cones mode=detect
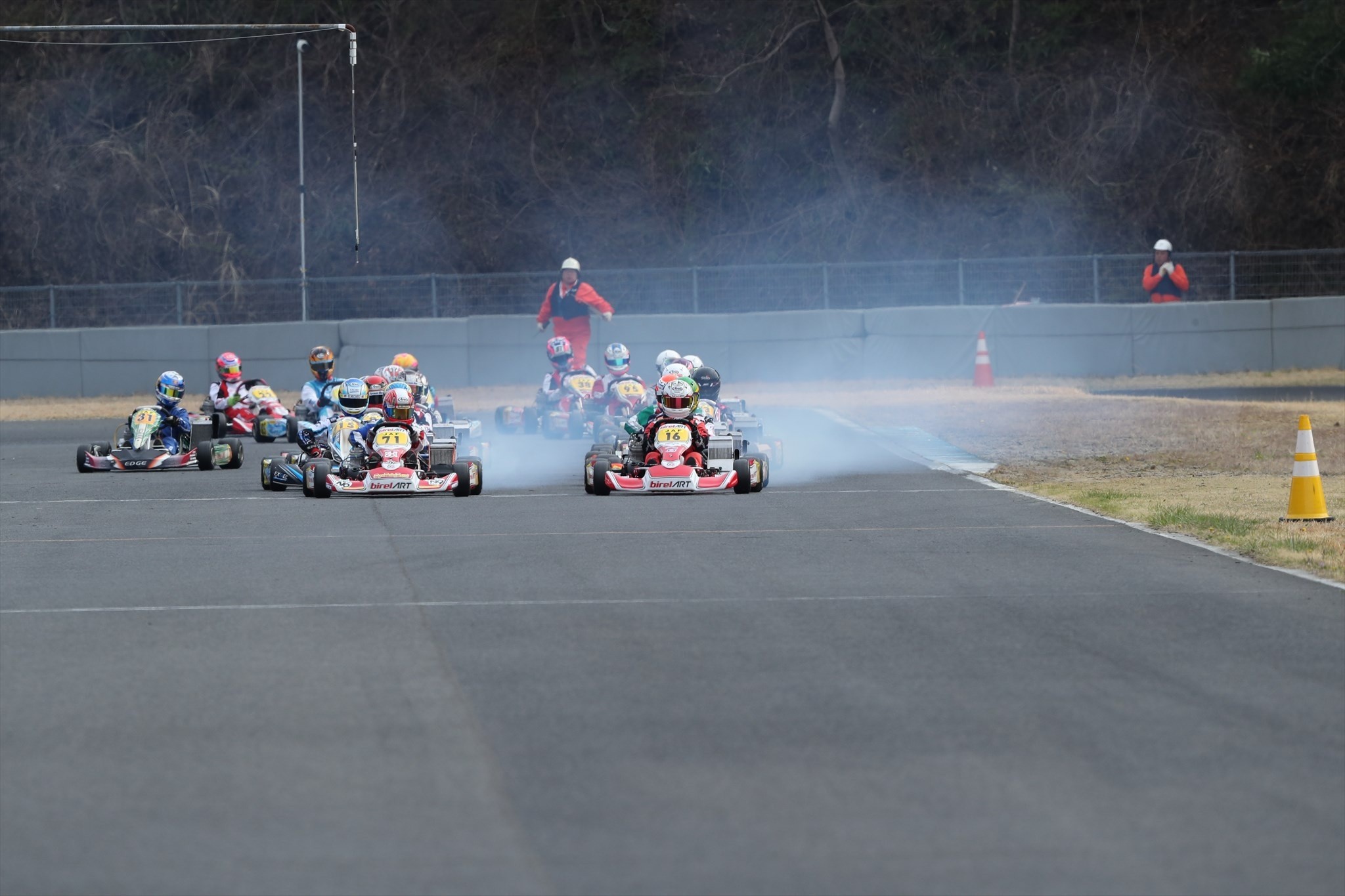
[970,330,996,387]
[1277,413,1335,523]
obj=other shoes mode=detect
[153,439,164,449]
[122,441,133,450]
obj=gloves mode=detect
[650,427,656,441]
[537,323,545,332]
[602,311,612,322]
[227,394,241,406]
[368,430,376,443]
[721,413,727,422]
[604,390,614,400]
[691,424,700,442]
[409,430,419,443]
[165,415,180,427]
[1159,262,1175,276]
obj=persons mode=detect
[592,343,732,476]
[542,335,597,412]
[537,257,615,365]
[121,371,191,455]
[1142,239,1189,303]
[300,344,443,469]
[209,352,292,423]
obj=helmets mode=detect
[604,343,631,375]
[1153,239,1172,252]
[338,378,369,418]
[655,349,703,410]
[382,365,404,384]
[382,381,412,401]
[547,336,574,371]
[382,388,415,426]
[692,367,721,401]
[374,367,384,377]
[397,370,423,399]
[155,371,185,407]
[561,257,580,273]
[392,353,418,371]
[215,352,242,383]
[363,376,388,409]
[309,346,335,381]
[661,380,694,418]
[359,376,368,381]
